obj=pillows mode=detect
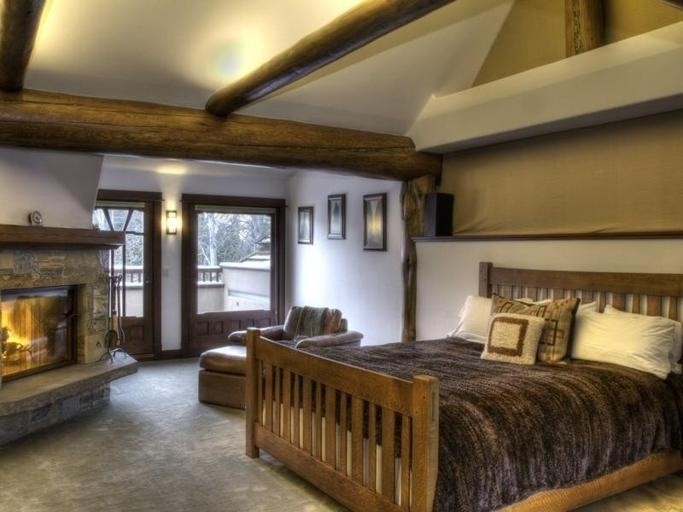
[490,295,579,365]
[448,300,554,345]
[570,312,675,381]
[523,297,598,314]
[478,314,546,367]
[605,304,683,375]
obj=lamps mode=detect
[167,211,177,234]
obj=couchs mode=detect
[198,305,364,409]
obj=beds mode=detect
[244,263,683,510]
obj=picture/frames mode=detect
[297,205,312,244]
[328,194,346,240]
[361,192,388,251]
